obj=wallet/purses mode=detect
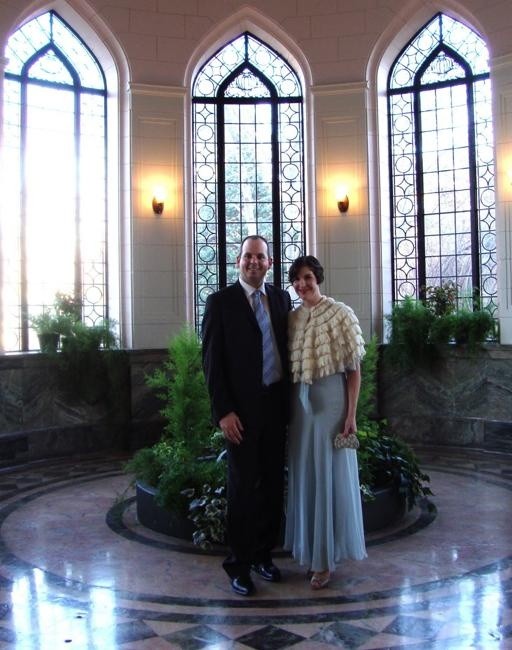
[334,433,359,449]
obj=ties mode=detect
[254,291,274,386]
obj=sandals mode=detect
[311,571,330,589]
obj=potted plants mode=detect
[385,282,497,368]
[120,329,438,551]
[27,310,78,353]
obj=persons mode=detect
[281,256,369,589]
[201,236,293,595]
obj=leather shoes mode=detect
[231,574,254,596]
[251,563,281,581]
